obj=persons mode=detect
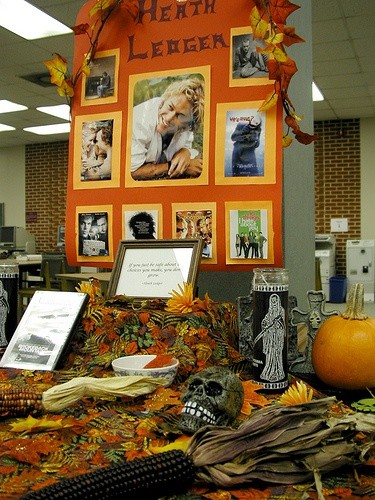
[95,72,110,98]
[81,121,112,180]
[176,212,212,258]
[235,230,267,260]
[230,116,263,176]
[231,35,268,78]
[130,79,204,180]
[78,212,108,255]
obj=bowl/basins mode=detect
[112,355,179,388]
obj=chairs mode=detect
[17,261,68,325]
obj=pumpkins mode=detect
[311,283,375,390]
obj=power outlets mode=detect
[331,218,348,232]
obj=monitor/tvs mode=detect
[0,226,35,250]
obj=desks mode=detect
[56,272,113,291]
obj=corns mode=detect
[0,389,43,417]
[18,449,196,500]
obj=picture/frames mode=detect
[214,100,276,186]
[125,64,211,188]
[105,238,204,302]
[75,204,113,262]
[80,47,120,106]
[224,201,275,265]
[172,202,217,265]
[229,26,276,88]
[73,111,122,190]
[122,204,163,240]
